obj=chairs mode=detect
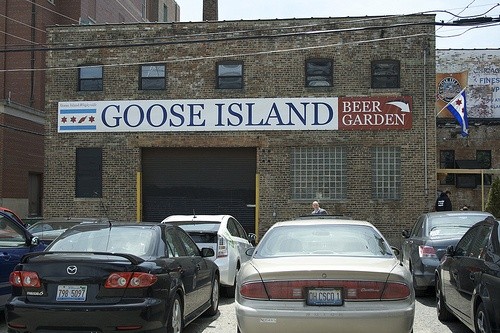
[280,238,303,252]
[91,236,111,252]
[330,235,362,253]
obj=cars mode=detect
[233,213,416,333]
[159,213,257,299]
[0,206,118,314]
[433,216,500,333]
[399,210,496,298]
[4,220,221,333]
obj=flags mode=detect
[446,90,470,137]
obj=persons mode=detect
[435,190,453,211]
[312,201,328,215]
